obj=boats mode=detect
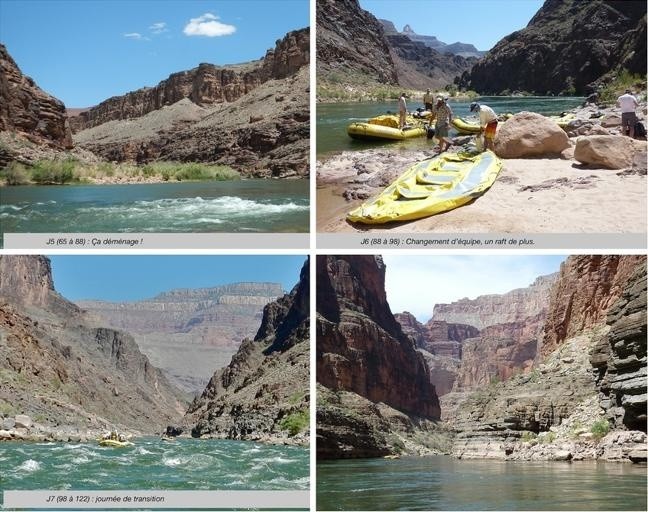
[450,111,578,134]
[101,438,132,448]
[348,107,451,138]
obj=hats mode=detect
[436,96,443,101]
[470,102,476,112]
[401,92,408,97]
[427,88,431,92]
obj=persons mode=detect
[423,88,433,112]
[616,88,641,138]
[398,92,408,127]
[469,101,498,151]
[429,97,453,154]
[101,427,127,443]
